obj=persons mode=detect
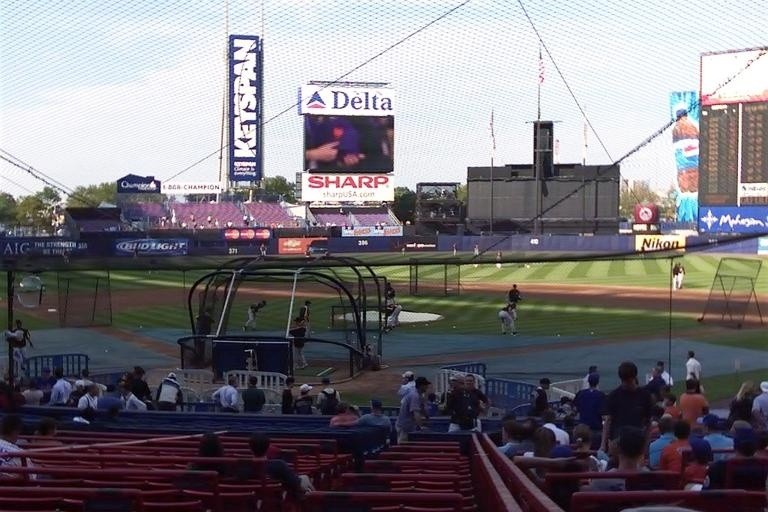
[305,116,393,172]
[672,264,682,291]
[289,317,309,370]
[678,264,686,289]
[195,306,215,355]
[498,303,519,336]
[509,284,522,321]
[10,320,32,378]
[299,301,311,339]
[384,281,395,308]
[242,300,266,332]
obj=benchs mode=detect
[72,199,396,232]
[1,407,480,511]
[513,446,768,512]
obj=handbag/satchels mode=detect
[78,395,95,422]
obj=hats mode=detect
[367,399,383,410]
[721,419,753,438]
[696,414,719,426]
[759,380,768,393]
[587,372,600,385]
[448,373,464,384]
[415,376,432,386]
[300,384,313,392]
[166,371,176,378]
[402,371,415,378]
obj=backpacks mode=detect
[320,388,339,416]
